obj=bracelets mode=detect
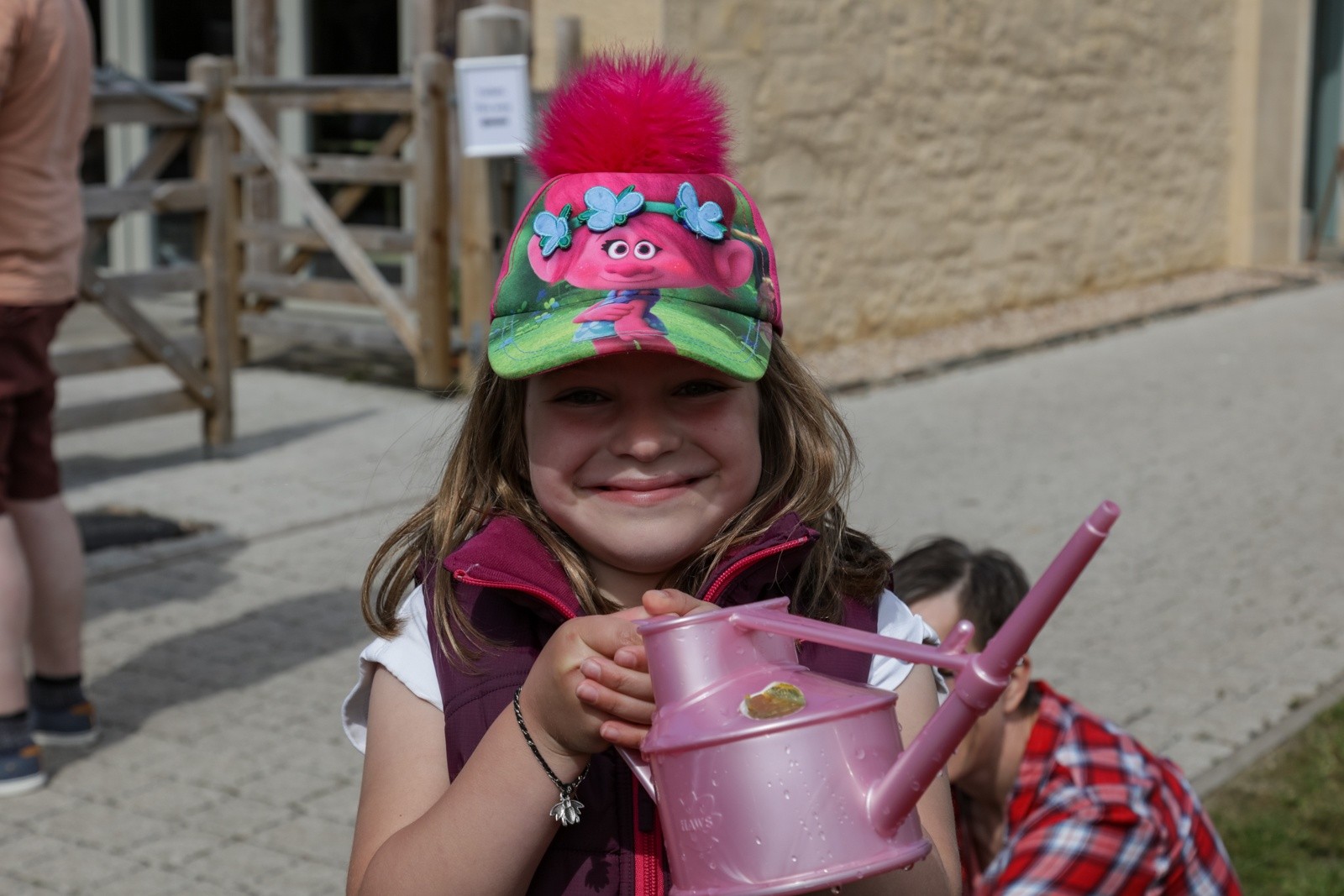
[514,687,595,825]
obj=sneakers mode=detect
[26,694,103,748]
[0,730,51,800]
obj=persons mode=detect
[0,0,104,797]
[341,63,961,896]
[891,534,1243,896]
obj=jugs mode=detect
[611,498,1124,896]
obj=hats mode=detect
[486,32,784,380]
